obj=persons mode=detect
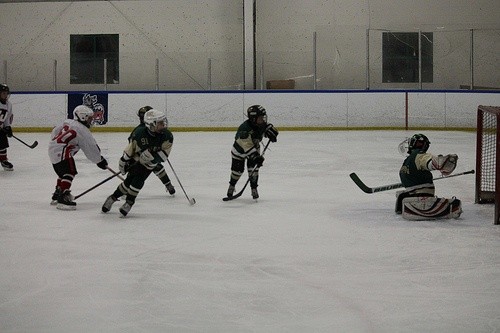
[0,83,14,169]
[48,104,108,207]
[394,133,463,220]
[226,105,279,199]
[102,105,175,217]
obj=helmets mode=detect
[137,105,167,129]
[0,83,10,92]
[406,134,431,154]
[247,104,266,119]
[73,105,94,122]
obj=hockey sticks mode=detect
[5,133,38,150]
[108,165,125,181]
[349,169,475,194]
[222,139,270,202]
[71,171,121,199]
[167,159,196,207]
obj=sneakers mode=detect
[1,161,14,171]
[227,184,235,197]
[250,187,260,203]
[118,200,133,218]
[49,185,77,210]
[166,183,176,198]
[101,195,120,214]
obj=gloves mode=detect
[249,152,265,168]
[119,153,131,175]
[4,126,12,137]
[264,124,279,142]
[142,149,168,170]
[97,156,108,170]
